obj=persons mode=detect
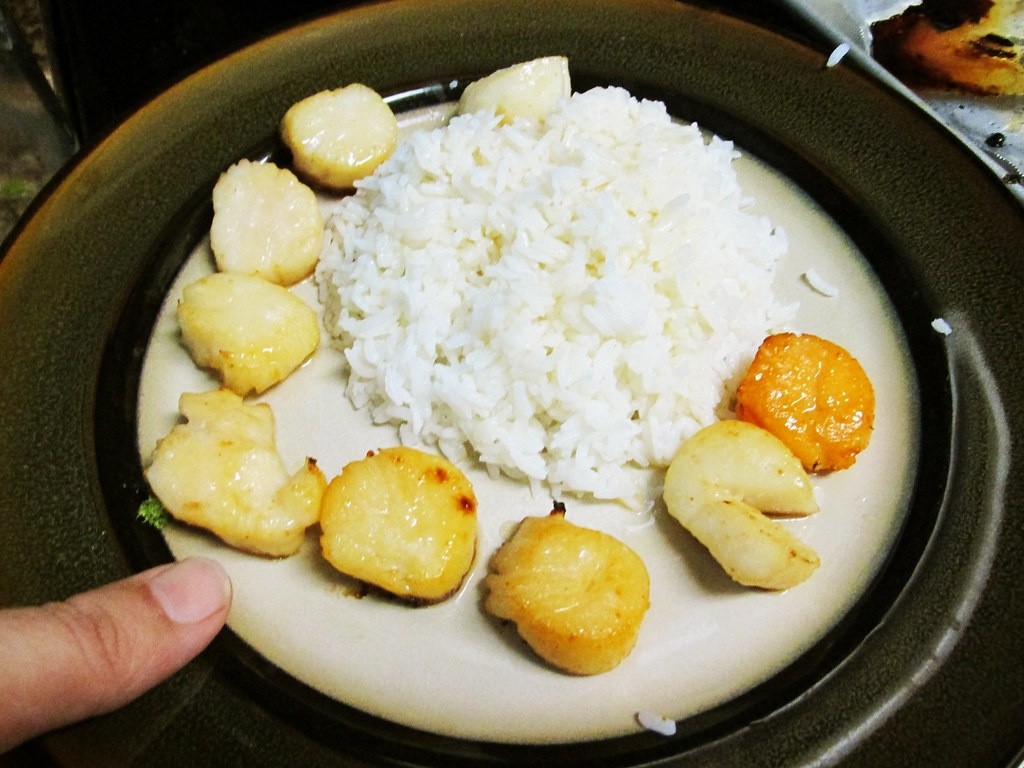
[0,556,232,747]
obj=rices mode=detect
[314,80,835,522]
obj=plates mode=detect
[0,0,1024,768]
